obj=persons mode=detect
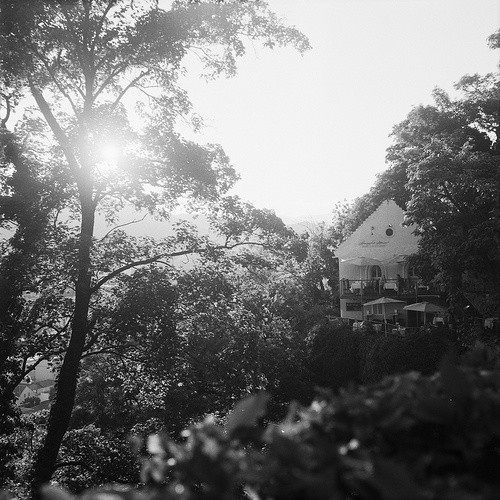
[396,273,404,291]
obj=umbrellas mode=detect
[388,254,407,261]
[362,297,403,305]
[403,301,447,325]
[341,257,382,266]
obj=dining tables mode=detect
[351,278,399,296]
